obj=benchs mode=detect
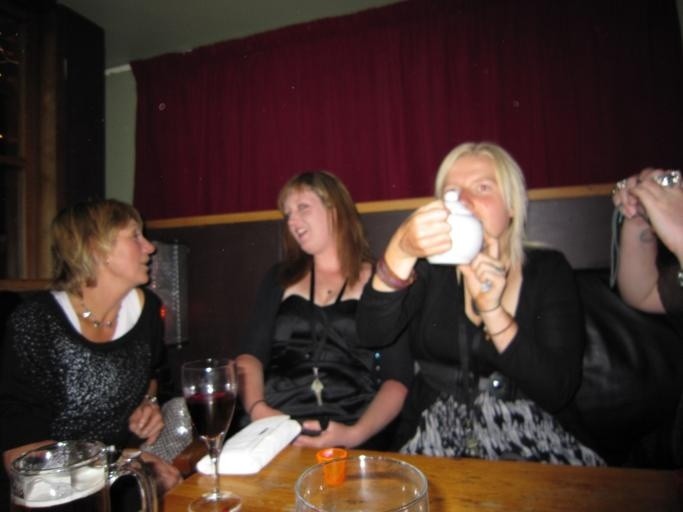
[143,182,631,431]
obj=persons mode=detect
[353,143,609,467]
[612,168,683,316]
[0,198,185,495]
[233,169,415,454]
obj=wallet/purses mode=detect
[195,414,303,476]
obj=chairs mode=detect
[1,272,68,307]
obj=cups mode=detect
[317,447,348,484]
[293,456,432,512]
[9,436,160,512]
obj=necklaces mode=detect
[78,299,121,329]
[308,264,348,406]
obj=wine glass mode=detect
[181,356,240,512]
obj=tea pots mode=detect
[411,191,483,267]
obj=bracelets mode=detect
[485,318,514,340]
[376,256,416,290]
[248,399,266,419]
[144,394,158,404]
[478,304,501,313]
